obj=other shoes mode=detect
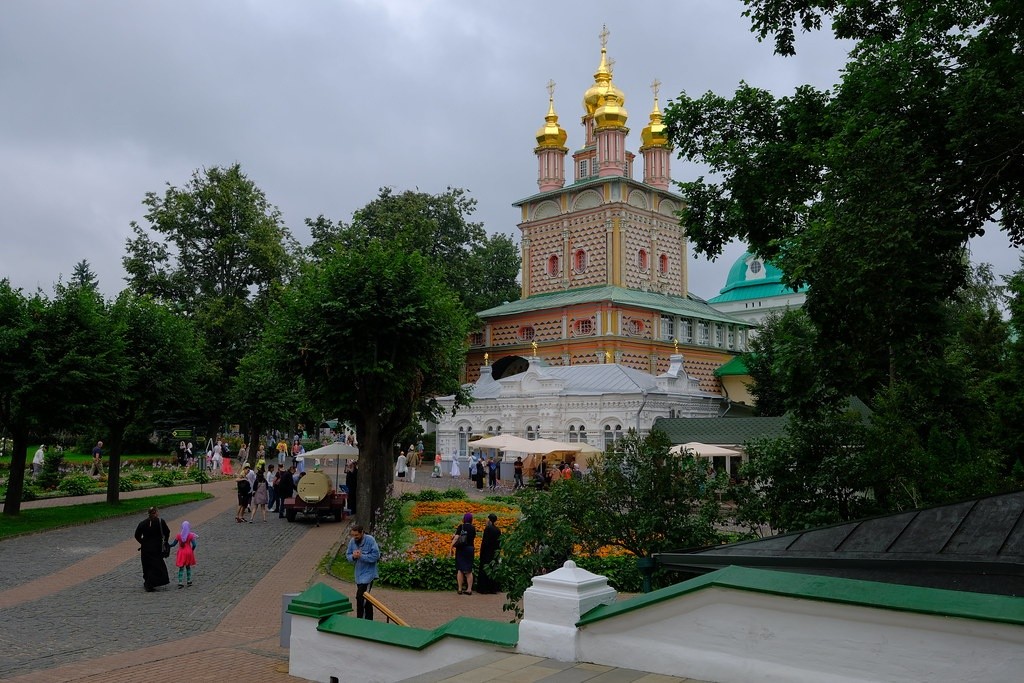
[187,581,193,587]
[249,519,254,522]
[177,584,184,589]
[279,515,286,518]
[272,509,279,513]
[235,517,247,522]
[457,590,462,595]
[263,520,267,524]
[463,591,472,595]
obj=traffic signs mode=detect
[196,437,205,442]
[172,429,193,439]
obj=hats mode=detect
[410,444,414,450]
[245,463,250,468]
[574,463,579,468]
[517,457,522,459]
[255,469,264,476]
[463,513,472,523]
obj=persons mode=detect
[31,444,47,479]
[178,432,357,524]
[135,506,170,591]
[346,525,380,620]
[89,441,108,479]
[266,429,267,431]
[479,513,502,595]
[395,440,424,483]
[431,449,443,478]
[450,513,476,596]
[467,451,581,492]
[451,449,461,479]
[168,521,198,590]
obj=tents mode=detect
[661,441,742,457]
[466,434,605,486]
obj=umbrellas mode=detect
[296,441,358,493]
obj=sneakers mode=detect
[267,507,274,511]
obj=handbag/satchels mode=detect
[296,455,303,461]
[395,470,398,477]
[161,540,170,557]
[472,465,478,475]
[453,524,467,547]
[407,462,410,467]
